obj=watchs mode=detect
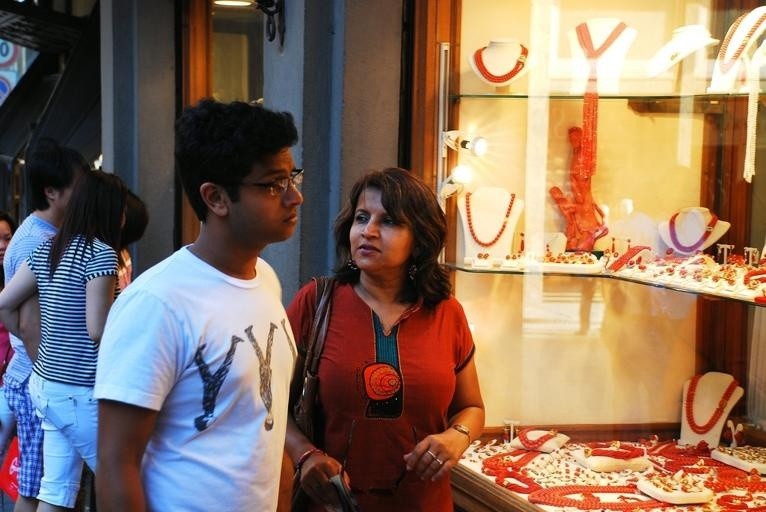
[448,423,472,444]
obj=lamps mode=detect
[444,128,491,159]
[440,164,477,191]
[212,0,285,49]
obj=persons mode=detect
[282,168,486,511]
[90,94,305,511]
[0,146,150,512]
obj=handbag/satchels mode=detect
[293,371,320,512]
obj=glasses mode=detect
[238,169,304,197]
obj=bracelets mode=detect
[295,448,324,480]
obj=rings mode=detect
[436,458,443,465]
[428,450,436,459]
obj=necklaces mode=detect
[576,19,628,58]
[481,376,766,510]
[719,13,766,73]
[669,212,719,253]
[474,44,528,82]
[465,192,516,247]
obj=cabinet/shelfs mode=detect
[431,0,766,512]
